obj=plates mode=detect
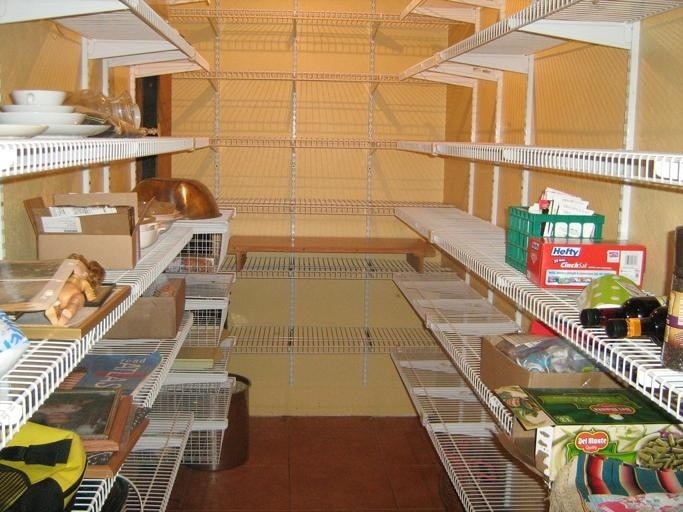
[0,104,112,139]
[634,432,683,469]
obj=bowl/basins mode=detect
[131,178,223,220]
[10,90,68,105]
[138,201,183,251]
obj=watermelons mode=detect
[575,451,682,503]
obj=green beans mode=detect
[638,434,683,471]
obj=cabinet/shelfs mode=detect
[159,4,473,393]
[387,0,681,511]
[0,2,240,510]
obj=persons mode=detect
[37,399,88,426]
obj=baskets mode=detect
[503,205,606,276]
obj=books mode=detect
[50,354,162,397]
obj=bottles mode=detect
[580,295,669,329]
[605,305,669,347]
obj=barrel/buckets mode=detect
[189,373,251,473]
